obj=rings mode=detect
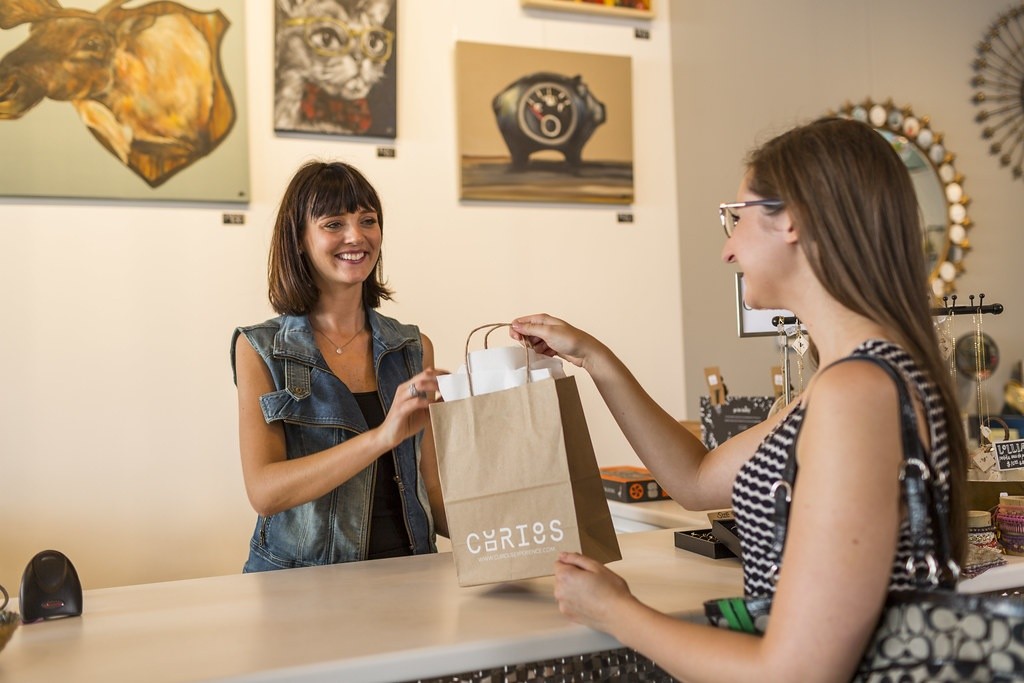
[408,383,418,395]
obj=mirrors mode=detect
[827,99,975,309]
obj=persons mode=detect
[231,159,451,575]
[509,115,972,683]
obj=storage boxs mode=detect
[595,467,672,504]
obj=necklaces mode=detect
[311,323,365,356]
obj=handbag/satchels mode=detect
[700,355,1024,683]
[427,322,623,587]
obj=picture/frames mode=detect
[735,272,809,336]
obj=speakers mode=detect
[18,549,83,624]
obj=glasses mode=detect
[720,199,783,238]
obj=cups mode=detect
[967,511,992,528]
[1000,496,1024,513]
